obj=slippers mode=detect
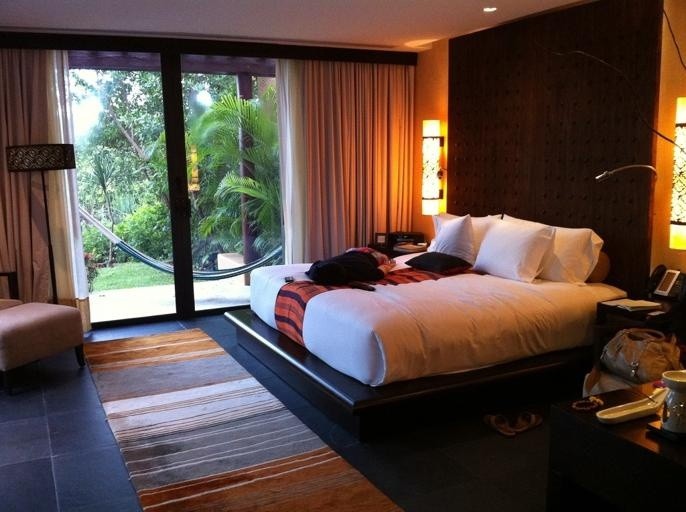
[509,406,543,432]
[483,412,516,437]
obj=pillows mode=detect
[405,214,604,285]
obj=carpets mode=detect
[81,327,404,512]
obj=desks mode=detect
[545,372,686,512]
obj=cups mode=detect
[660,370,685,434]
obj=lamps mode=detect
[6,144,76,303]
[595,164,657,184]
[668,98,686,251]
[422,119,445,217]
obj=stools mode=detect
[0,301,86,396]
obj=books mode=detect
[392,244,428,253]
[618,299,661,312]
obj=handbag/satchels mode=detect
[600,327,681,384]
[582,367,638,399]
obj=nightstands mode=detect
[367,241,430,259]
[596,296,686,359]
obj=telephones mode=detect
[647,264,684,298]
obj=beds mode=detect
[225,248,625,437]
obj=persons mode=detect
[306,246,396,286]
